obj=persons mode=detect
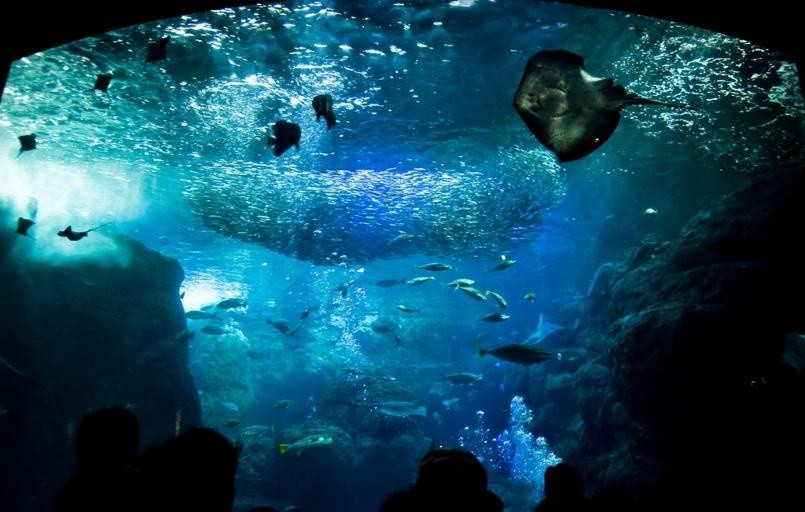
[53,407,805,512]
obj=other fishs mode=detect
[175,145,566,458]
